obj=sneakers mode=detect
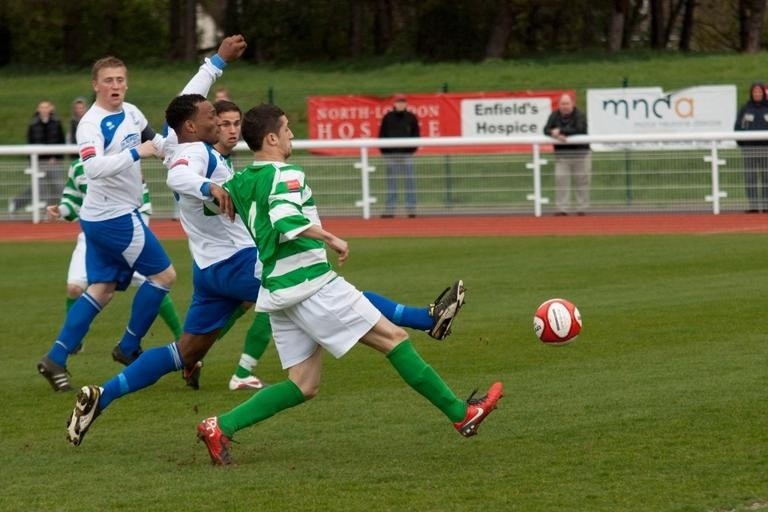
[66,385,105,448]
[35,353,75,393]
[111,342,142,367]
[451,381,505,439]
[424,278,468,341]
[227,373,264,393]
[181,360,204,391]
[195,415,237,467]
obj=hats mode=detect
[393,94,407,103]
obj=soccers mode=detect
[533,298,582,346]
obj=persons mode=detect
[543,93,591,216]
[10,102,67,219]
[195,104,503,465]
[732,81,768,213]
[377,93,420,219]
[72,99,88,145]
[38,56,177,390]
[45,158,185,339]
[176,99,273,390]
[64,36,468,447]
